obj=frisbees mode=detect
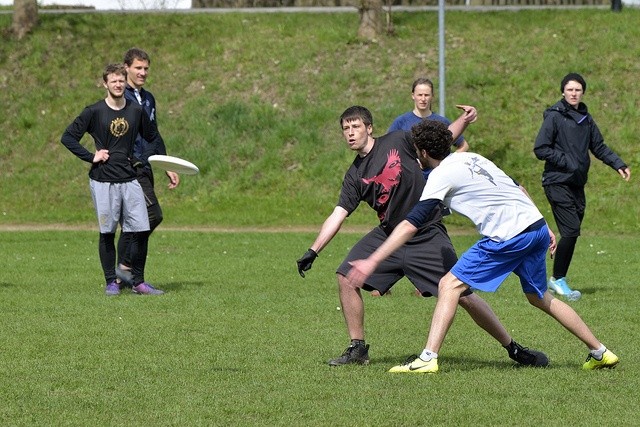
[147,155,199,175]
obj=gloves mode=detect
[296,247,318,278]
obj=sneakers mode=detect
[388,353,439,375]
[582,347,619,370]
[508,340,548,369]
[131,281,164,294]
[105,278,120,295]
[327,341,370,366]
[548,274,573,295]
[115,263,135,288]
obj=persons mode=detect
[534,73,631,296]
[118,50,164,286]
[346,120,620,374]
[297,105,549,364]
[368,80,470,297]
[62,61,164,296]
